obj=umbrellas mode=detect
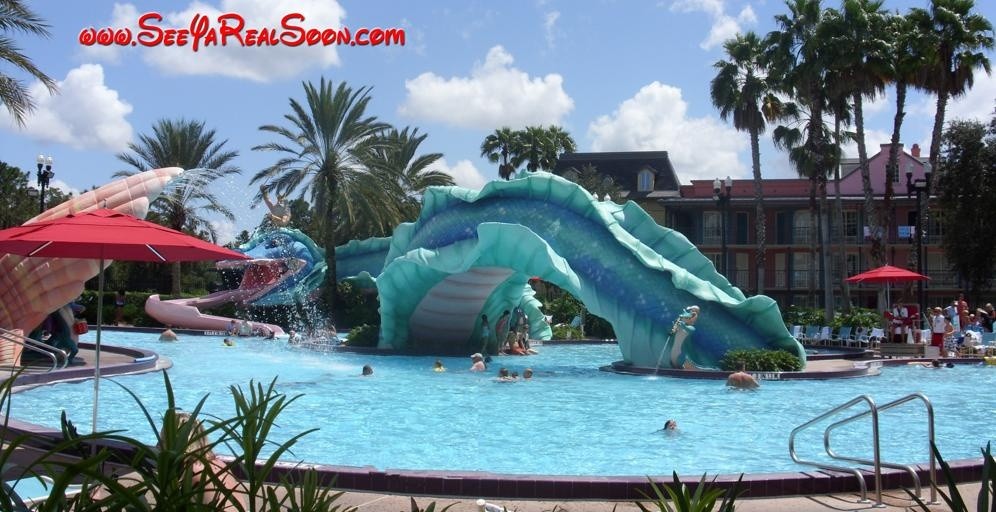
[1,198,255,432]
[843,261,932,311]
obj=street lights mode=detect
[36,153,56,213]
[903,159,935,329]
[711,175,734,283]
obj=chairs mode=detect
[791,325,884,351]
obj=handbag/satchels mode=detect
[904,316,913,326]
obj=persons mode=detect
[664,420,678,430]
[223,317,339,348]
[889,293,996,367]
[111,288,126,326]
[469,309,540,382]
[432,360,449,373]
[363,365,374,375]
[726,359,760,389]
[158,323,179,342]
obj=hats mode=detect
[471,352,483,359]
[953,300,958,306]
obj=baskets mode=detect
[73,319,88,335]
